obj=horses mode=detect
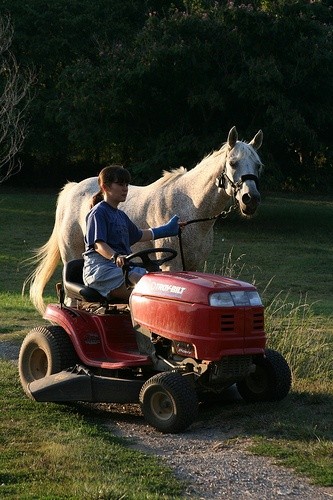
[18,126,263,316]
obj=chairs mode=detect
[63,259,130,314]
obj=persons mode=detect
[83,165,188,302]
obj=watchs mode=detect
[111,252,119,264]
